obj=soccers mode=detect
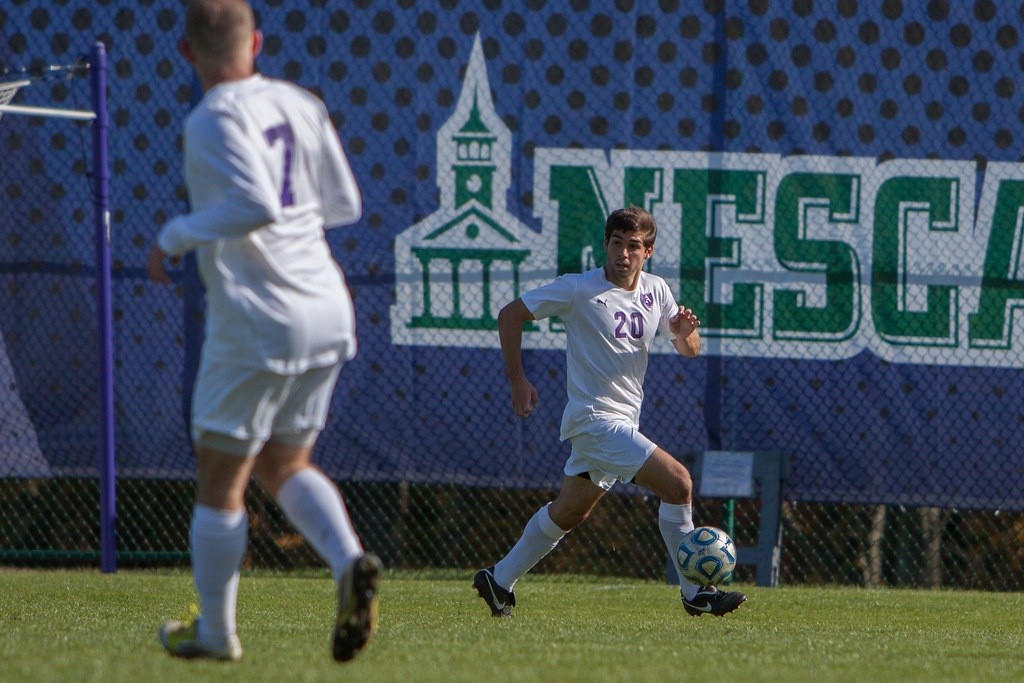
[676,525,738,586]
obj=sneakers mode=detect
[470,565,516,618]
[156,602,243,662]
[329,552,385,663]
[679,581,748,618]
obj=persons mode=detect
[472,207,748,619]
[145,0,382,664]
